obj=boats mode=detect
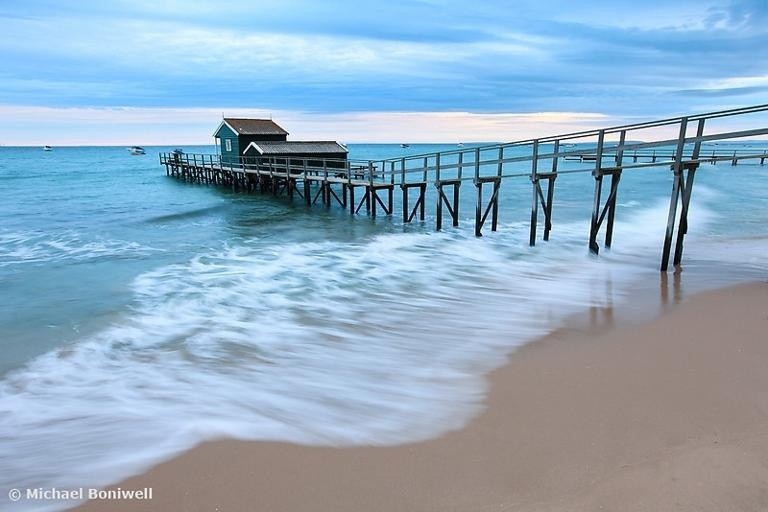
[44,147,52,152]
[128,147,144,155]
